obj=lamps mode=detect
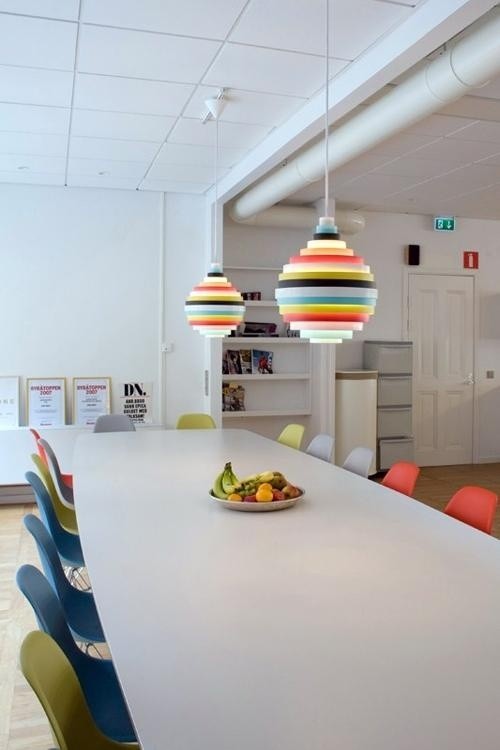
[182,85,245,340]
[273,0,381,348]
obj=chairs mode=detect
[177,414,215,429]
[92,414,138,434]
[16,427,141,750]
[442,486,499,534]
[277,423,422,496]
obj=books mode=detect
[223,349,273,412]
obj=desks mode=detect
[74,428,499,750]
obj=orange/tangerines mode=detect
[228,493,243,501]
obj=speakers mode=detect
[408,244,420,266]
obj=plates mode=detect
[209,489,304,512]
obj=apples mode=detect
[272,485,299,501]
[243,496,256,502]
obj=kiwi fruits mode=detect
[272,471,287,490]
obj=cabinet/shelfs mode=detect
[331,370,380,477]
[221,264,314,418]
[361,340,418,476]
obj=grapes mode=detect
[240,485,256,498]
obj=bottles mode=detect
[252,291,261,300]
[244,292,251,300]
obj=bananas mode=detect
[241,470,274,485]
[213,462,242,499]
